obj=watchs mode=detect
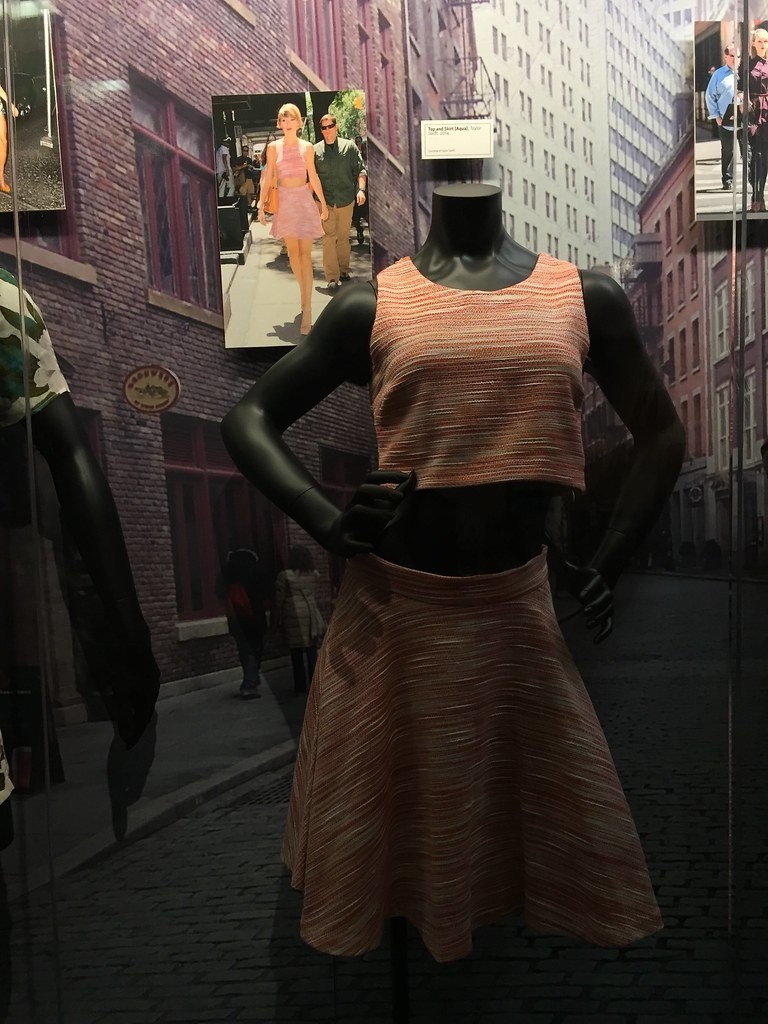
[356,187,366,194]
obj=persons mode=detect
[257,103,329,336]
[705,63,722,138]
[225,557,268,702]
[736,28,768,212]
[219,183,686,967]
[216,136,235,196]
[705,45,753,191]
[0,84,20,192]
[352,136,369,244]
[275,545,328,693]
[0,269,161,1024]
[307,113,366,293]
[252,150,262,195]
[231,143,255,210]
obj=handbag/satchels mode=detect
[310,607,326,638]
[264,142,279,214]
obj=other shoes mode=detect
[752,195,765,212]
[300,317,311,335]
[339,272,350,281]
[242,690,261,699]
[329,281,338,290]
[723,183,732,190]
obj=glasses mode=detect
[321,123,335,130]
[243,150,249,152]
